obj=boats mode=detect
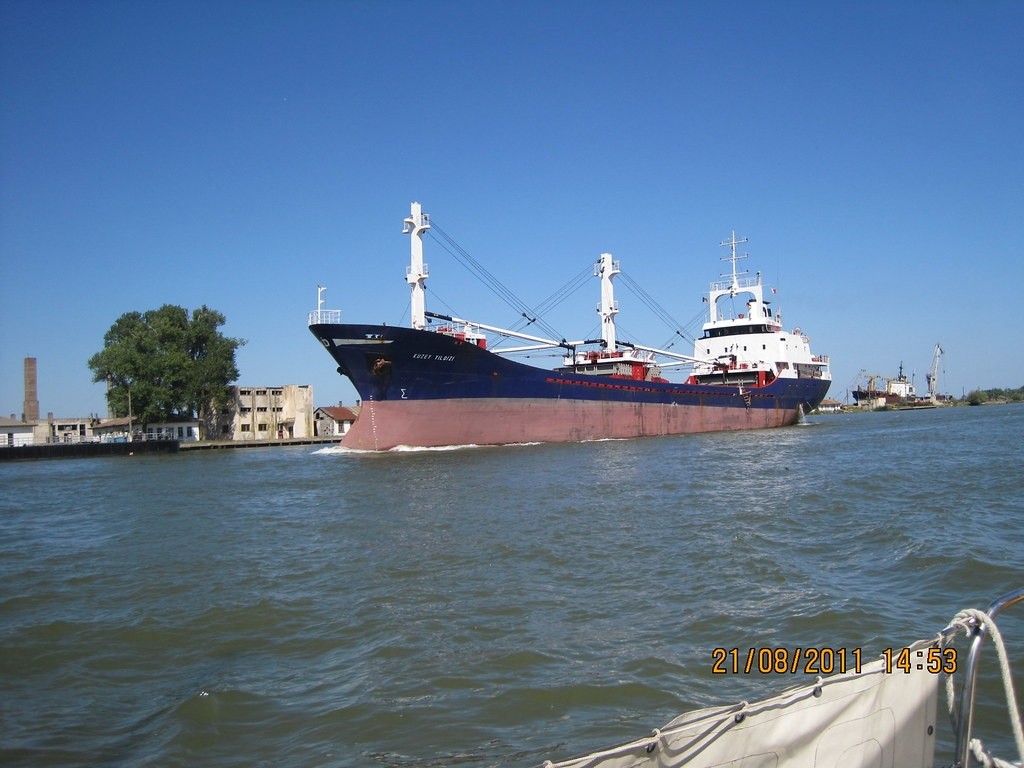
[308,201,831,457]
[532,586,1023,768]
[849,342,949,405]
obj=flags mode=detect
[702,297,709,303]
[771,288,779,294]
